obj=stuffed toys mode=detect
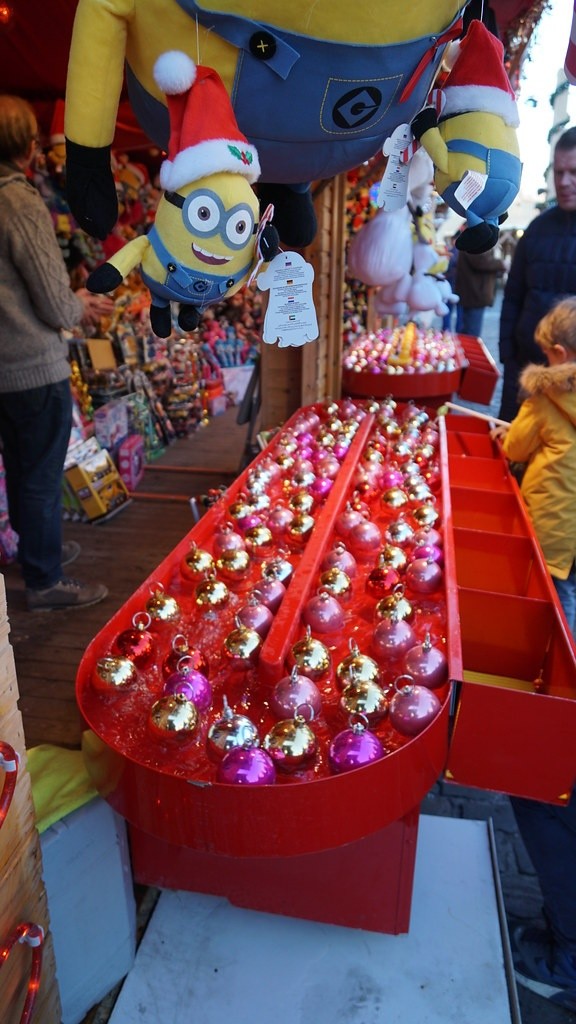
[66,0,524,361]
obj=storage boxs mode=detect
[63,426,134,526]
[220,365,259,405]
[118,435,145,492]
[94,399,129,468]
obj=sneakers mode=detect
[506,923,575,1010]
[61,541,81,566]
[27,576,109,613]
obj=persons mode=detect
[442,128,576,1011]
[0,98,116,613]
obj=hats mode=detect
[150,51,262,192]
[440,19,516,118]
